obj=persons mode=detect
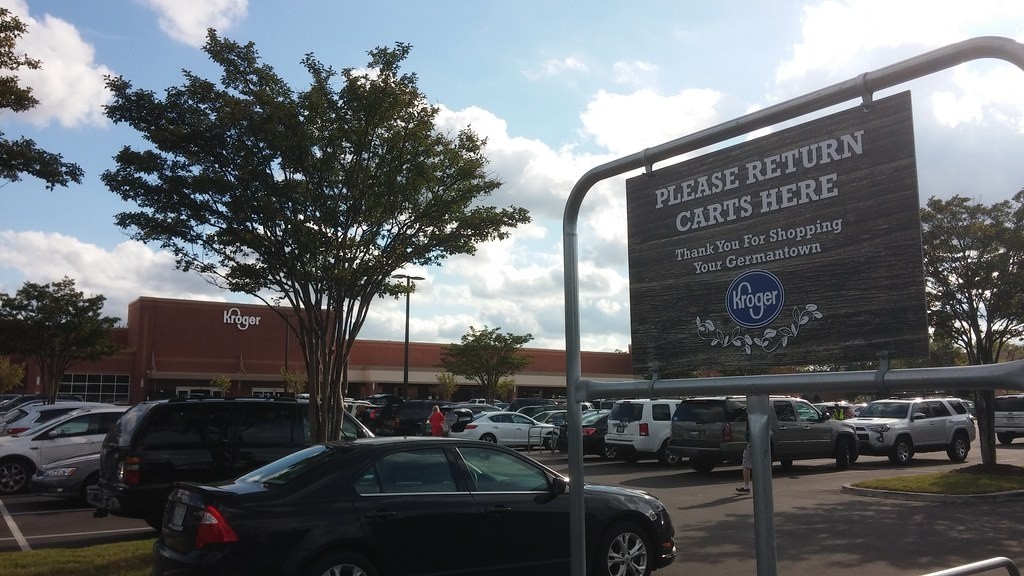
[833,404,843,419]
[429,405,444,436]
[735,420,752,494]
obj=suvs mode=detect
[3,403,117,436]
[995,392,1024,444]
[366,393,450,435]
[604,397,687,467]
[510,398,559,413]
[86,396,450,531]
[667,395,860,475]
[844,395,976,464]
[0,395,85,411]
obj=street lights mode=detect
[283,313,297,397]
[392,275,424,397]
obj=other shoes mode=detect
[736,486,750,494]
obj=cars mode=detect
[32,453,102,505]
[267,391,881,460]
[0,405,132,493]
[153,436,677,576]
